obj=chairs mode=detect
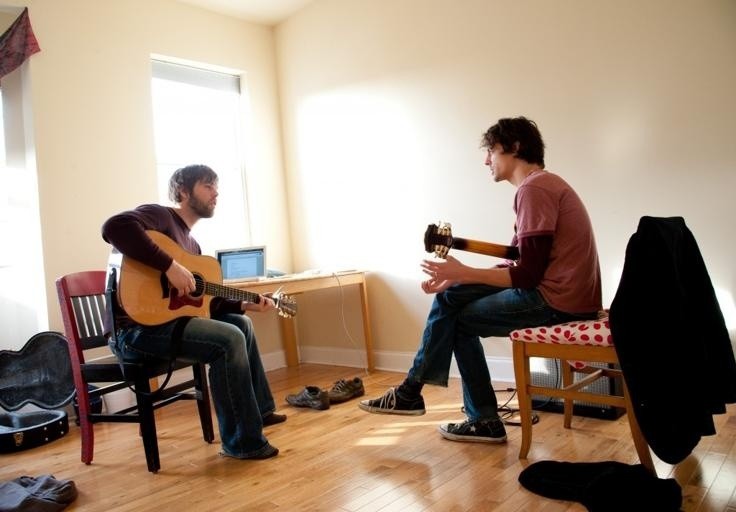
[54,269,216,475]
[510,215,685,476]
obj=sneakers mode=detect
[359,387,425,416]
[286,385,329,410]
[329,378,364,403]
[438,420,508,444]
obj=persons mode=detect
[354,114,606,447]
[99,165,290,461]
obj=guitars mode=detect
[105,230,299,326]
[423,221,520,261]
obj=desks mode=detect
[223,271,378,373]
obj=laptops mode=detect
[216,246,269,284]
